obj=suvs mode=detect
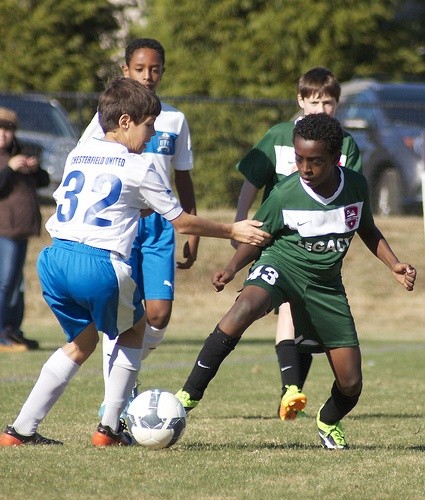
[332,80,425,216]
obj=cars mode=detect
[0,90,99,208]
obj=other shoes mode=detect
[0,334,40,351]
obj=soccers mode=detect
[126,389,187,449]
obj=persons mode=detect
[172,112,417,449]
[0,75,274,447]
[75,38,201,420]
[0,107,51,352]
[231,68,362,420]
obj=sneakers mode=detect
[0,425,63,448]
[175,388,199,414]
[129,379,141,405]
[277,385,307,421]
[90,418,136,448]
[97,401,132,418]
[317,403,348,450]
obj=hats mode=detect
[0,108,17,128]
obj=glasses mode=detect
[0,122,16,130]
